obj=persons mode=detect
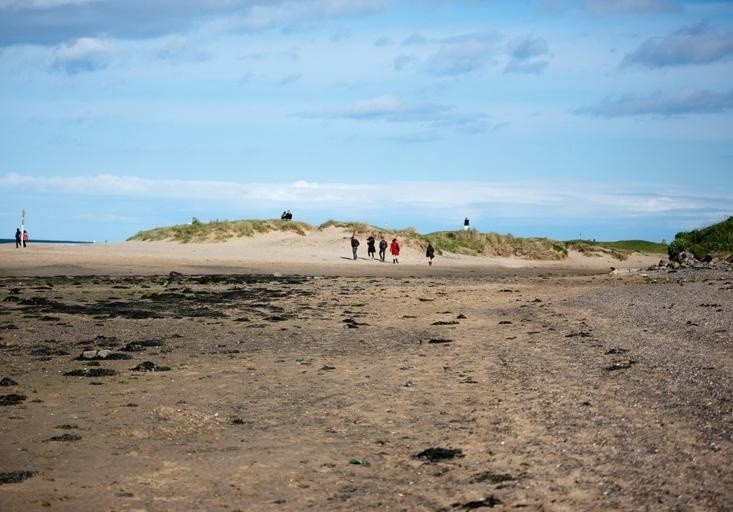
[286,209,292,221]
[281,211,286,220]
[349,236,360,260]
[426,243,435,266]
[390,238,400,263]
[366,236,376,261]
[378,236,388,262]
[464,217,469,231]
[15,228,22,248]
[23,230,28,247]
